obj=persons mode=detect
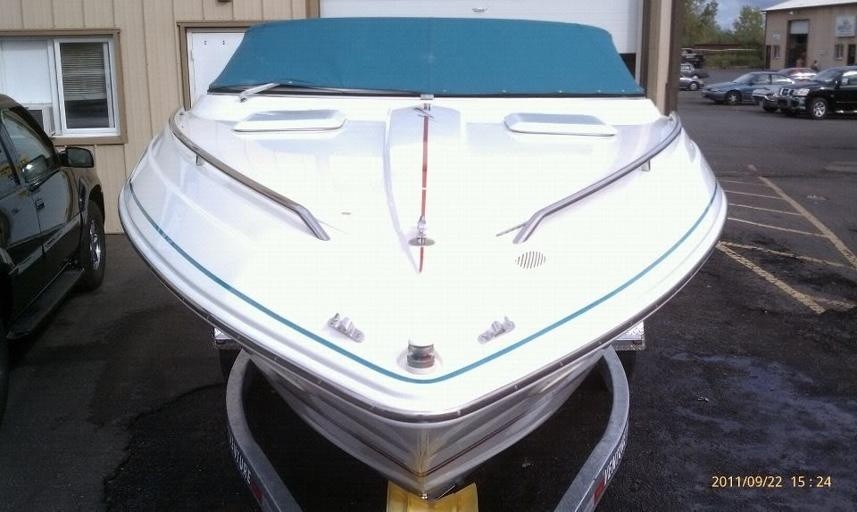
[811,59,818,71]
[796,51,807,70]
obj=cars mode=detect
[701,66,857,119]
[679,48,707,91]
[0,94,105,355]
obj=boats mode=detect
[117,15,728,503]
